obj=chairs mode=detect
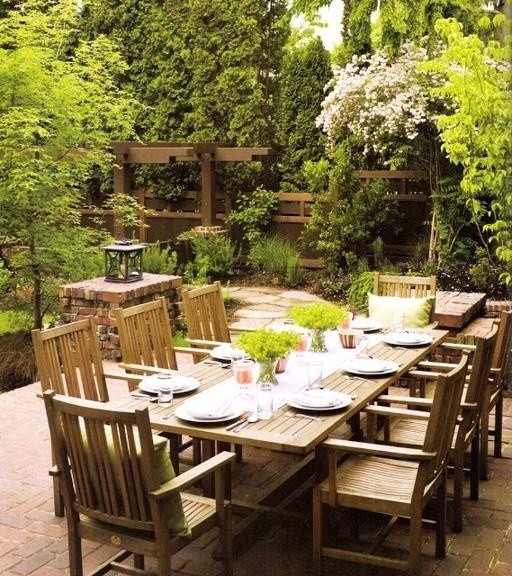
[113,296,232,485]
[313,349,469,576]
[408,310,511,478]
[42,390,236,576]
[182,280,243,463]
[372,272,439,331]
[30,315,178,518]
[363,318,502,534]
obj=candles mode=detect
[117,264,126,279]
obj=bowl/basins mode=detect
[338,329,362,348]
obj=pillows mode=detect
[64,430,191,539]
[366,290,435,336]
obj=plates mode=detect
[344,359,400,376]
[384,329,432,347]
[288,388,352,411]
[138,375,200,393]
[349,317,383,332]
[175,399,243,424]
[210,351,248,362]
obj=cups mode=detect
[231,356,257,388]
[159,374,173,405]
[393,310,405,334]
[358,340,371,359]
[258,383,273,418]
[307,365,322,389]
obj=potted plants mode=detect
[286,301,350,351]
[236,329,304,389]
